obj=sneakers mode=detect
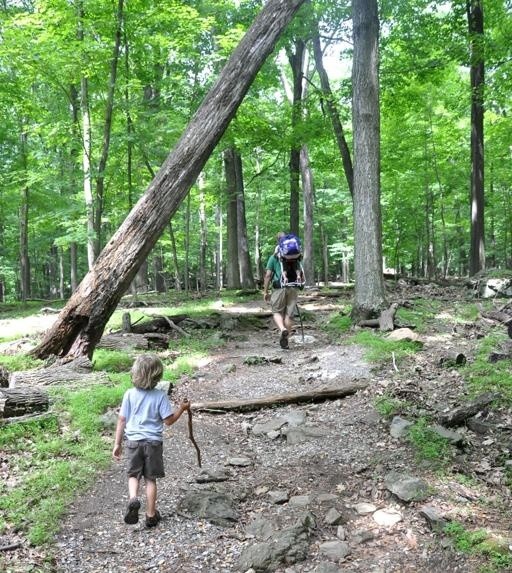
[146,510,162,527]
[124,497,141,524]
[280,328,288,346]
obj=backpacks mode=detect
[273,234,306,287]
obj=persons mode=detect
[263,249,307,349]
[273,231,302,283]
[112,352,189,527]
